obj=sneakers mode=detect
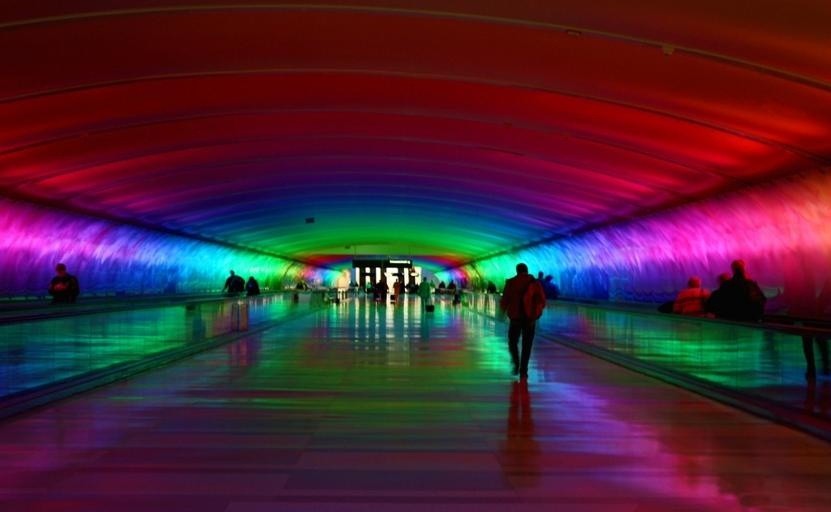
[512,367,528,380]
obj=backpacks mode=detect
[522,274,547,320]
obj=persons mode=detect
[292,271,560,312]
[48,263,82,302]
[246,276,260,298]
[720,258,768,324]
[499,263,548,378]
[223,269,246,295]
[672,274,712,318]
[701,271,734,318]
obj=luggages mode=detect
[390,295,396,301]
[425,297,435,313]
